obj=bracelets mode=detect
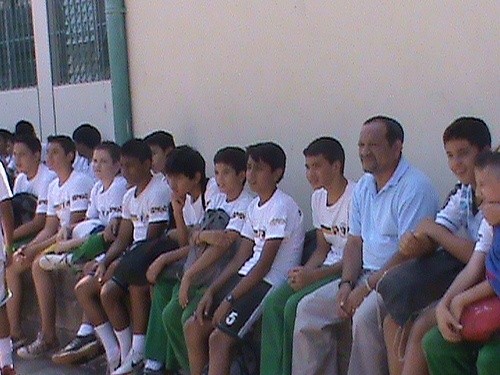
[338,279,356,288]
[365,276,372,292]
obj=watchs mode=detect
[225,291,236,305]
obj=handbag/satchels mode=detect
[179,208,239,299]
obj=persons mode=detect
[161,146,256,375]
[137,147,223,374]
[5,134,95,360]
[38,138,130,273]
[383,116,492,375]
[183,142,305,374]
[74,137,171,374]
[110,226,178,374]
[260,136,358,375]
[0,120,38,194]
[72,123,103,186]
[421,147,500,375]
[291,116,440,375]
[0,161,15,375]
[50,130,188,368]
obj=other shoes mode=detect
[52,334,101,364]
[142,358,163,375]
[108,350,121,375]
[7,335,28,354]
[112,348,148,375]
[17,334,62,360]
[39,254,74,270]
[0,362,16,375]
